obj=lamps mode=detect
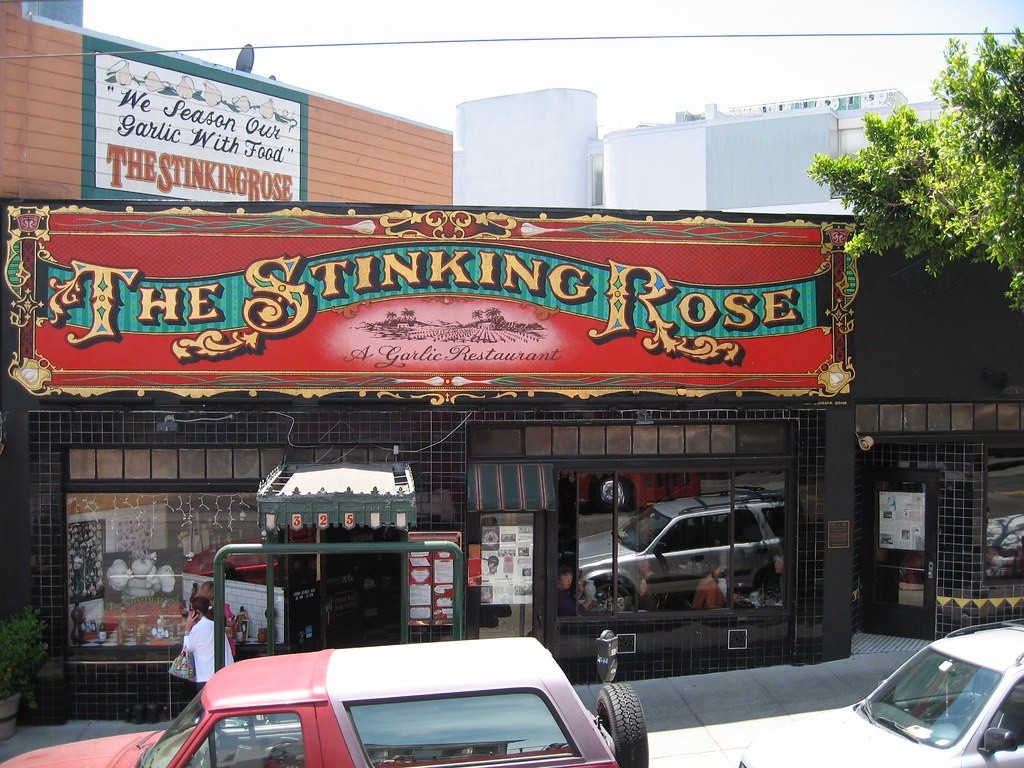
[155,414,181,434]
[391,445,410,474]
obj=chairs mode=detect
[656,580,692,611]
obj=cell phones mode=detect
[192,610,200,618]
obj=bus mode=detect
[578,468,701,513]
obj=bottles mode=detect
[236,605,249,643]
[761,584,766,608]
[70,602,84,642]
[136,626,142,645]
[98,616,107,643]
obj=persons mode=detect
[773,553,785,599]
[692,556,726,609]
[556,565,591,616]
[638,552,666,612]
[189,581,236,663]
[183,597,235,694]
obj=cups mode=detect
[258,628,267,642]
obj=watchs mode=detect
[185,631,189,634]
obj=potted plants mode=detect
[0,603,49,741]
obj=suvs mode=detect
[579,487,786,614]
[985,514,1024,576]
[180,524,317,617]
[738,618,1024,768]
[0,637,648,768]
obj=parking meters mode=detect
[595,630,619,684]
